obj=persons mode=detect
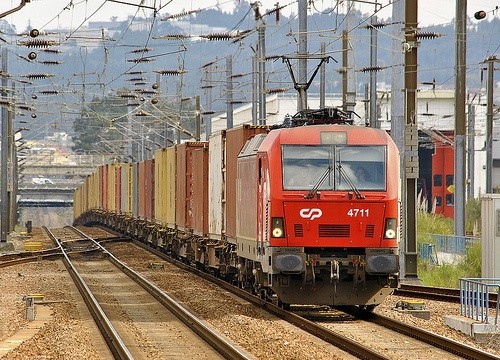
[352,167,383,189]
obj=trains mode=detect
[73,107,402,317]
